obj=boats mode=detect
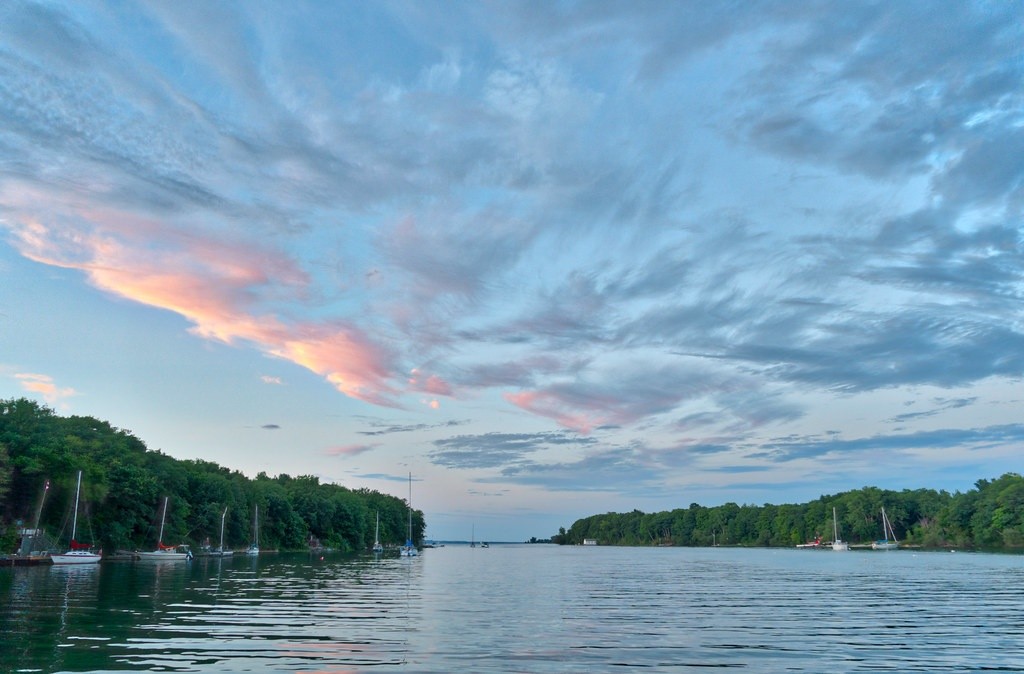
[106,553,140,561]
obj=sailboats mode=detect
[208,506,233,557]
[137,497,191,561]
[372,511,384,552]
[399,472,418,556]
[871,507,900,550]
[832,506,849,551]
[51,470,103,564]
[245,505,260,555]
